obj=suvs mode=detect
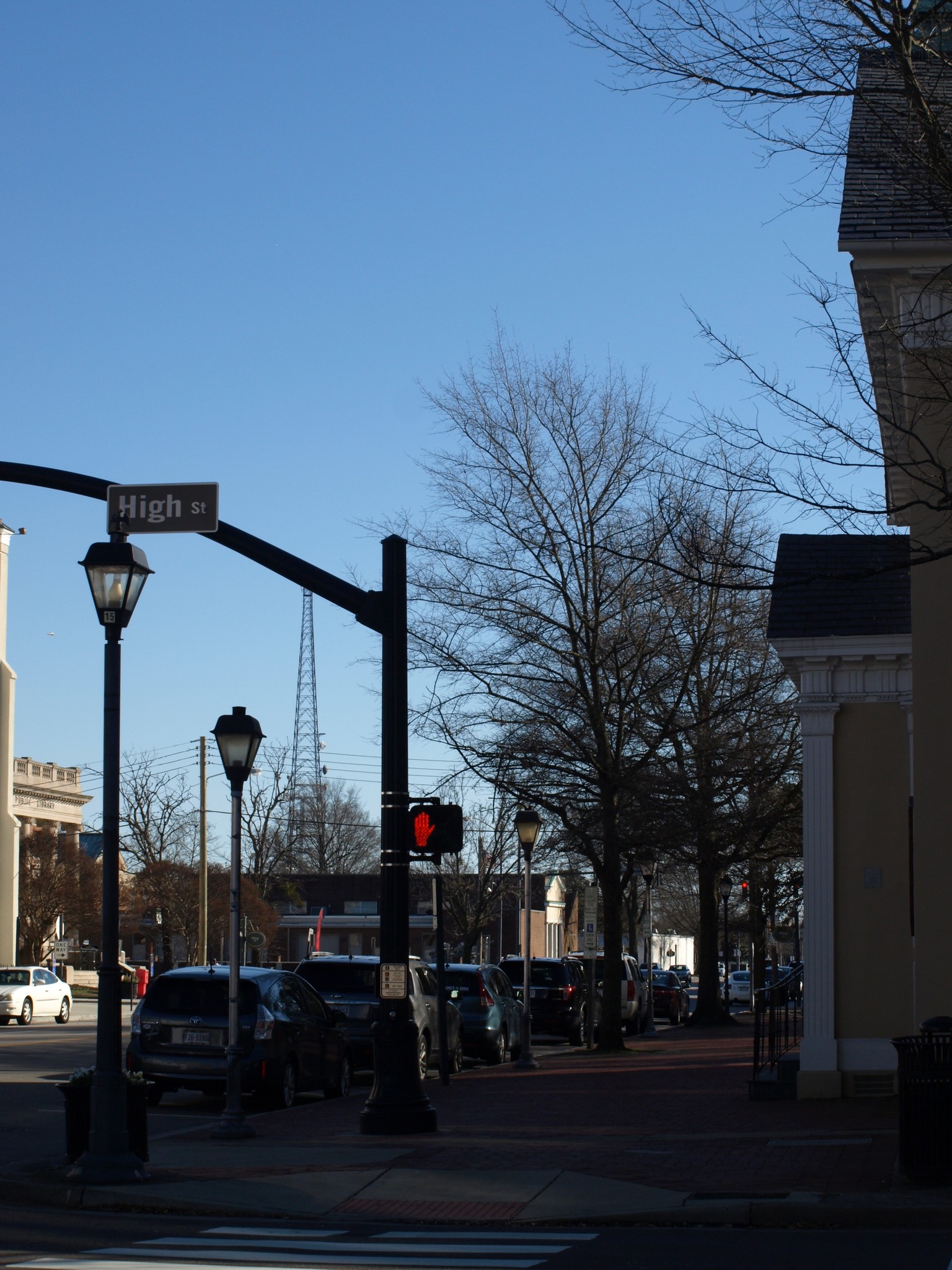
[562,950,649,1036]
[287,955,465,1084]
[426,961,527,1067]
[126,964,351,1107]
[498,952,592,1046]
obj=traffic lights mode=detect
[741,881,748,899]
[793,883,798,898]
[410,803,465,854]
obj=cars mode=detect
[639,969,691,1025]
[765,966,796,1002]
[721,970,751,1006]
[0,965,73,1025]
[788,960,804,983]
[717,963,725,977]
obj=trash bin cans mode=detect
[889,1034,952,1147]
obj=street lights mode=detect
[210,703,267,1138]
[719,872,734,1015]
[68,501,154,1185]
[511,805,546,1071]
[637,849,661,1041]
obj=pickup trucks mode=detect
[669,966,692,984]
